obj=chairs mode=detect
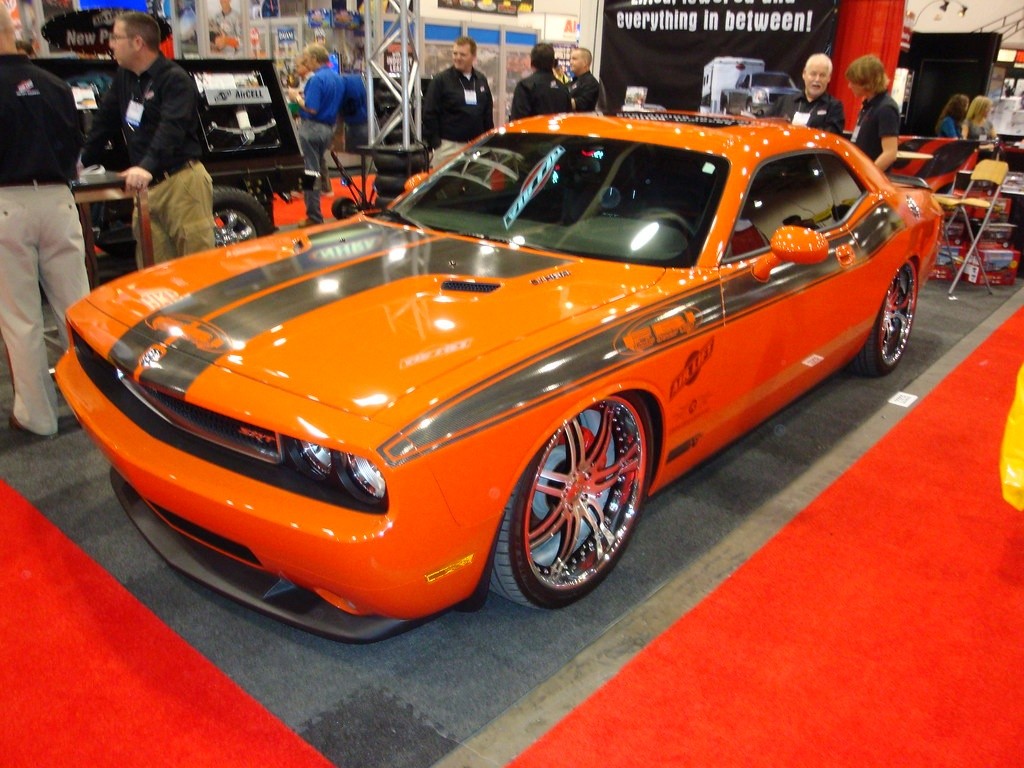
[932,159,1009,295]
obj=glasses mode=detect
[108,33,136,46]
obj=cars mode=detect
[718,70,804,122]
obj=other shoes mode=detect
[8,410,29,433]
[323,181,332,192]
[298,219,324,228]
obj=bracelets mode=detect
[295,95,301,104]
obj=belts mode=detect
[0,173,72,188]
[300,117,335,128]
[147,156,197,187]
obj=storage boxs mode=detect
[934,193,1021,287]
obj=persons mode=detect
[511,43,599,187]
[423,36,494,200]
[342,74,368,152]
[287,44,345,227]
[81,14,217,272]
[935,94,997,139]
[0,6,91,435]
[757,53,900,177]
[209,0,241,58]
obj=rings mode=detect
[137,182,143,190]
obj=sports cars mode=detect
[50,106,942,648]
